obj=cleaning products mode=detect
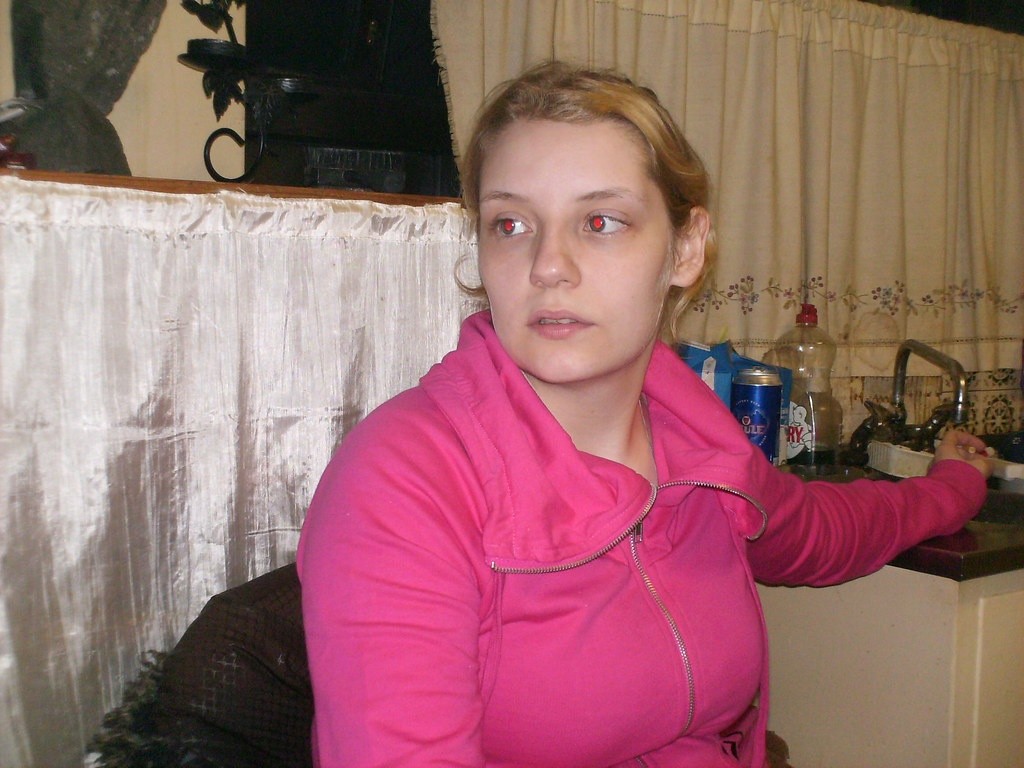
[767,302,845,476]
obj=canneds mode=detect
[730,365,783,465]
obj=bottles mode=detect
[774,303,843,478]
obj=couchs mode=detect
[150,562,793,768]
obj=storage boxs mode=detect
[678,338,794,466]
[870,441,934,478]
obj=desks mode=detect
[0,166,487,768]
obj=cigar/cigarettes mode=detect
[962,445,976,454]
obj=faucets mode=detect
[890,339,968,424]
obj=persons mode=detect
[295,60,993,768]
